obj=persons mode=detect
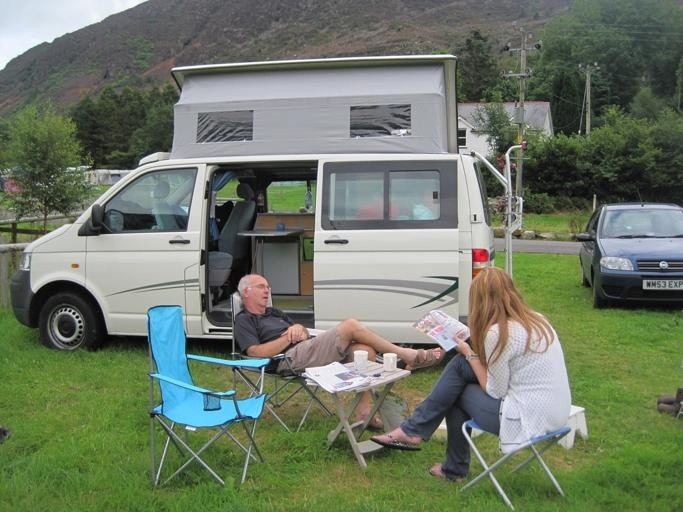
[370,266,570,485]
[235,274,445,430]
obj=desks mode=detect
[297,353,411,472]
[234,225,305,277]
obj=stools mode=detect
[558,403,588,448]
[460,415,576,510]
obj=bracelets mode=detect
[465,354,479,360]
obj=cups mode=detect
[277,223,284,231]
[382,352,397,371]
[353,350,368,371]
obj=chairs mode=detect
[225,284,333,438]
[216,182,255,261]
[150,178,233,301]
[144,302,275,494]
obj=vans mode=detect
[9,153,495,360]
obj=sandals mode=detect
[405,348,444,370]
[342,413,384,433]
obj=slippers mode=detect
[370,426,422,451]
[429,460,468,482]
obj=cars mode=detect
[574,201,683,309]
